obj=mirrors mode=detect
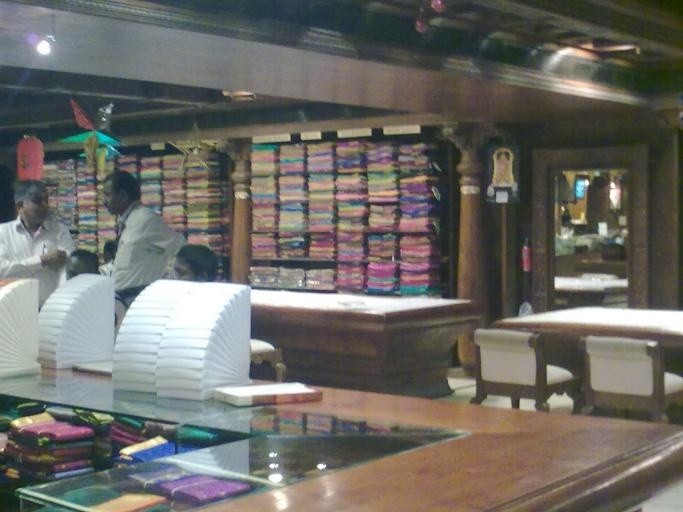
[530,143,652,313]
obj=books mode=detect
[214,382,324,408]
[74,363,113,380]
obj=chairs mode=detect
[576,333,683,425]
[249,346,287,383]
[468,328,584,414]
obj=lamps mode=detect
[36,15,60,56]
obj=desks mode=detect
[491,305,683,424]
[554,275,629,305]
[247,293,487,397]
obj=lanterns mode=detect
[16,134,44,182]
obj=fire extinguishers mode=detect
[522,235,531,273]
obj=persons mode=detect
[99,171,187,309]
[1,180,78,288]
[103,240,119,266]
[174,244,220,283]
[39,249,119,327]
